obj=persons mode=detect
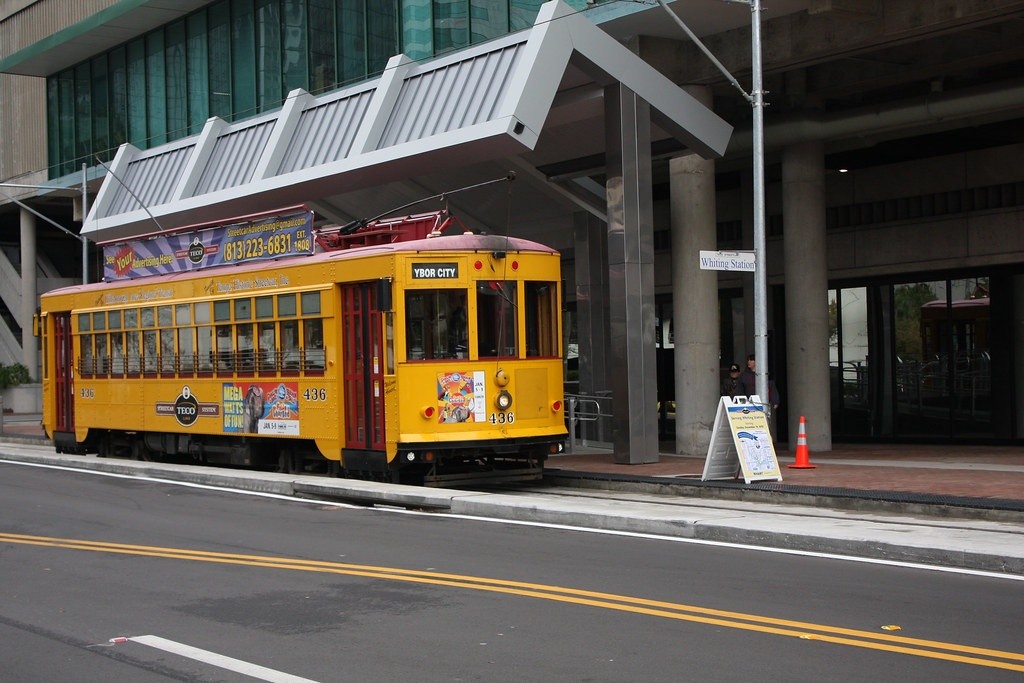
[720,364,746,396]
[737,355,779,409]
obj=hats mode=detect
[730,364,740,372]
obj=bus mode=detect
[31,160,574,486]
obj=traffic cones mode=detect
[787,416,819,469]
